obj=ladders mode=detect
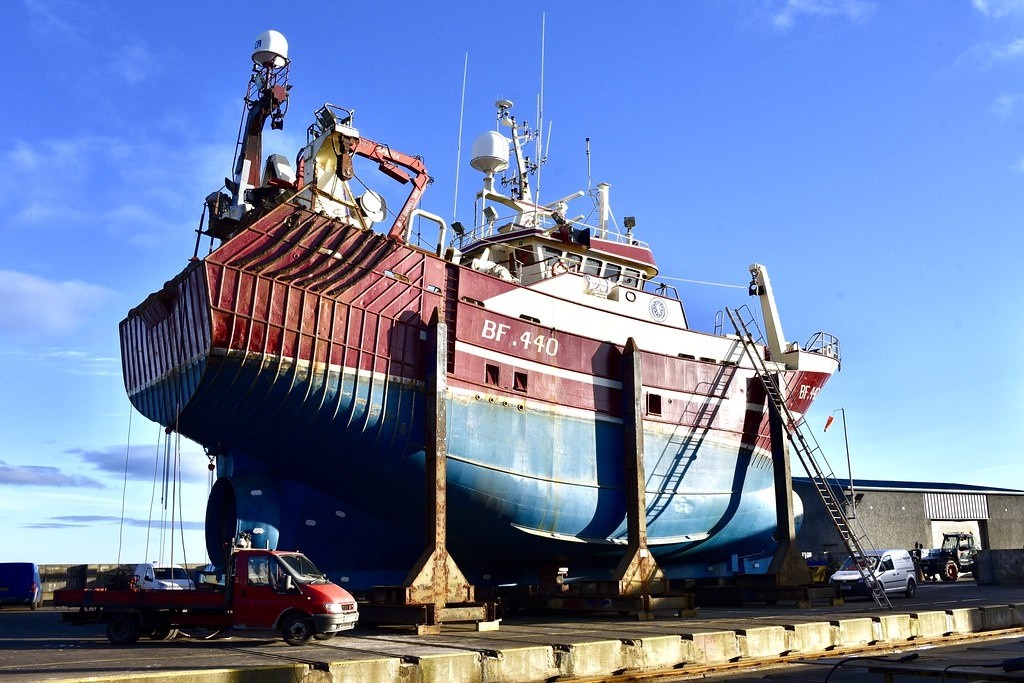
[726,304,891,608]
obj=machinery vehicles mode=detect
[919,532,979,584]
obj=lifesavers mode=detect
[552,261,570,276]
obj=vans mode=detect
[135,561,196,590]
[828,548,918,602]
[0,562,44,610]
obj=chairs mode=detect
[249,563,270,584]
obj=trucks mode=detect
[52,538,360,647]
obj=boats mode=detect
[119,14,841,592]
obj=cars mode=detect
[198,564,236,587]
